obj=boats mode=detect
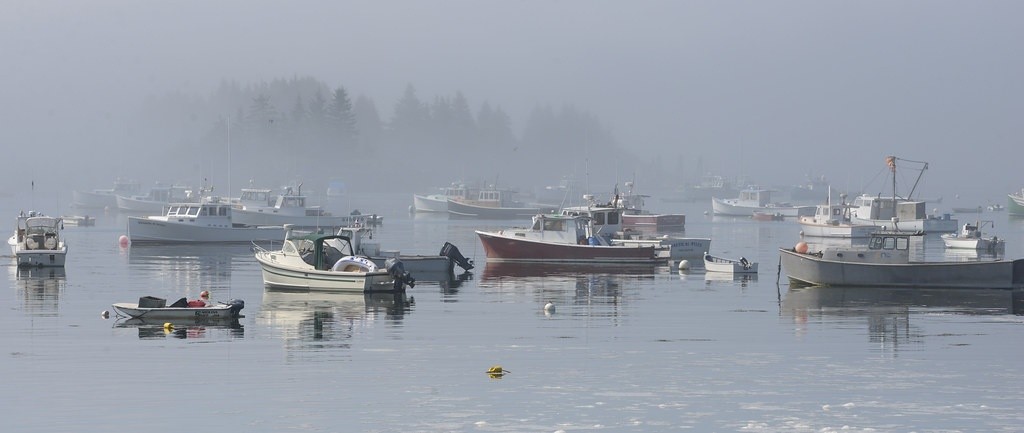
[558,155,711,265]
[9,262,66,318]
[661,175,741,202]
[705,271,758,283]
[798,155,958,239]
[250,224,416,293]
[782,275,1023,357]
[285,222,474,278]
[8,211,68,268]
[702,251,759,274]
[257,286,415,363]
[101,295,245,318]
[942,222,1006,251]
[710,185,818,219]
[473,209,668,267]
[477,258,658,289]
[66,115,386,248]
[776,229,1024,291]
[412,178,558,222]
[112,314,246,342]
